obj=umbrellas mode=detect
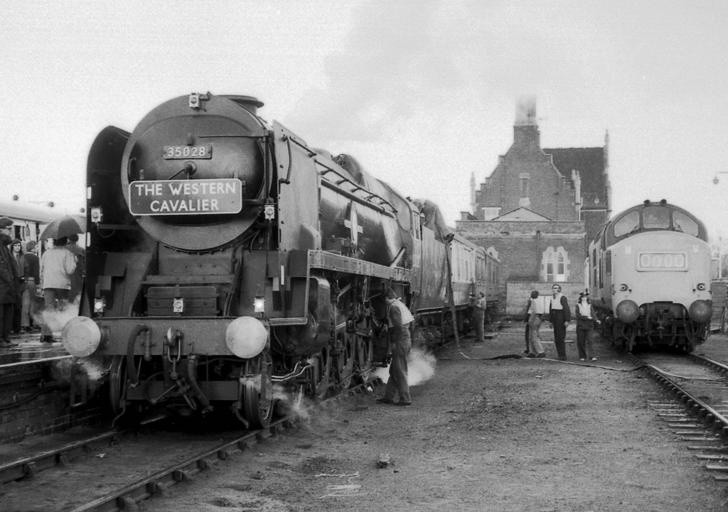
[40,215,86,242]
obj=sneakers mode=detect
[376,397,412,408]
[580,356,597,362]
[554,356,567,361]
[522,350,545,358]
[0,324,54,347]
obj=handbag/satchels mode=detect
[30,286,45,302]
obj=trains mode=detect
[588,200,713,354]
[60,92,505,430]
[0,192,89,328]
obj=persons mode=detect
[377,286,415,405]
[526,284,601,361]
[471,291,486,343]
[0,219,86,348]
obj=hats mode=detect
[9,239,20,245]
[0,217,14,228]
[579,288,589,296]
[26,240,37,252]
